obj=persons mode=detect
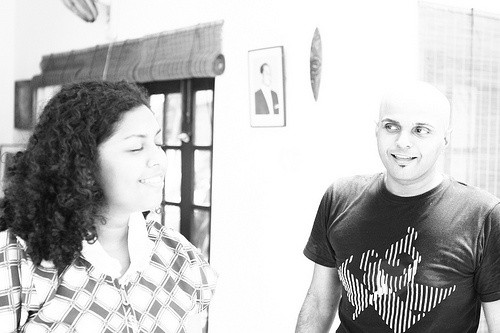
[0,78,219,333]
[294,80,500,333]
[255,63,280,115]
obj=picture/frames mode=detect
[247,45,286,126]
